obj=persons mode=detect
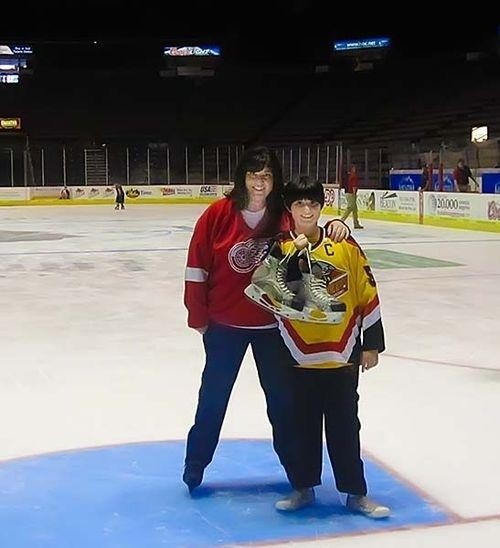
[451,158,480,192]
[250,172,392,520]
[180,143,352,491]
[114,183,125,210]
[338,165,363,229]
[419,165,432,192]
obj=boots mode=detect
[250,241,319,315]
[302,243,347,313]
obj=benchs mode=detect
[218,91,500,189]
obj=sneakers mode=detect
[275,488,315,511]
[345,494,390,518]
[182,464,204,488]
